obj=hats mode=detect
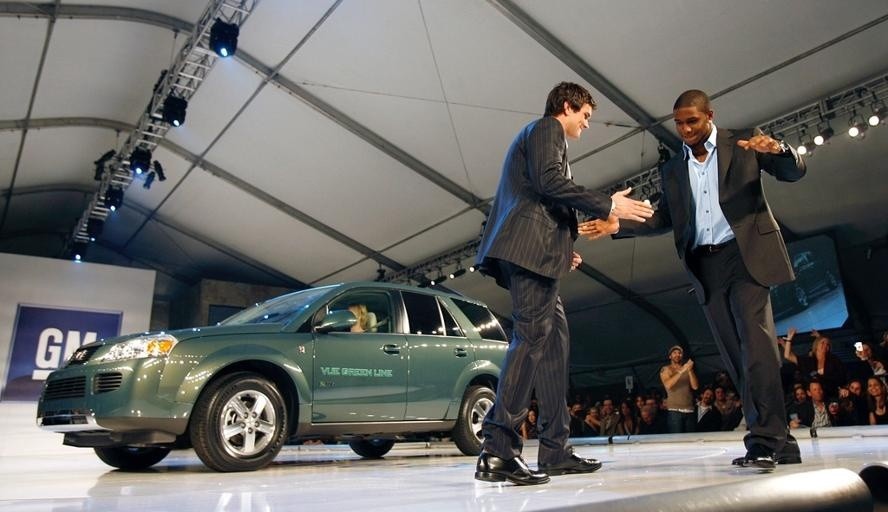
[668,345,684,360]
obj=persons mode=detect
[576,89,805,468]
[473,81,656,483]
[346,303,369,334]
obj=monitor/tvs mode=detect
[208,304,247,326]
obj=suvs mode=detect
[769,250,839,316]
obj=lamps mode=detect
[413,254,481,291]
[69,17,239,263]
[793,98,887,159]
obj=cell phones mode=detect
[855,341,863,352]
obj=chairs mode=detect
[365,312,377,333]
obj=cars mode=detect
[34,284,511,475]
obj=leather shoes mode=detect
[474,446,551,486]
[538,452,603,475]
[752,444,777,472]
[731,446,802,467]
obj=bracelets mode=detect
[608,199,615,217]
